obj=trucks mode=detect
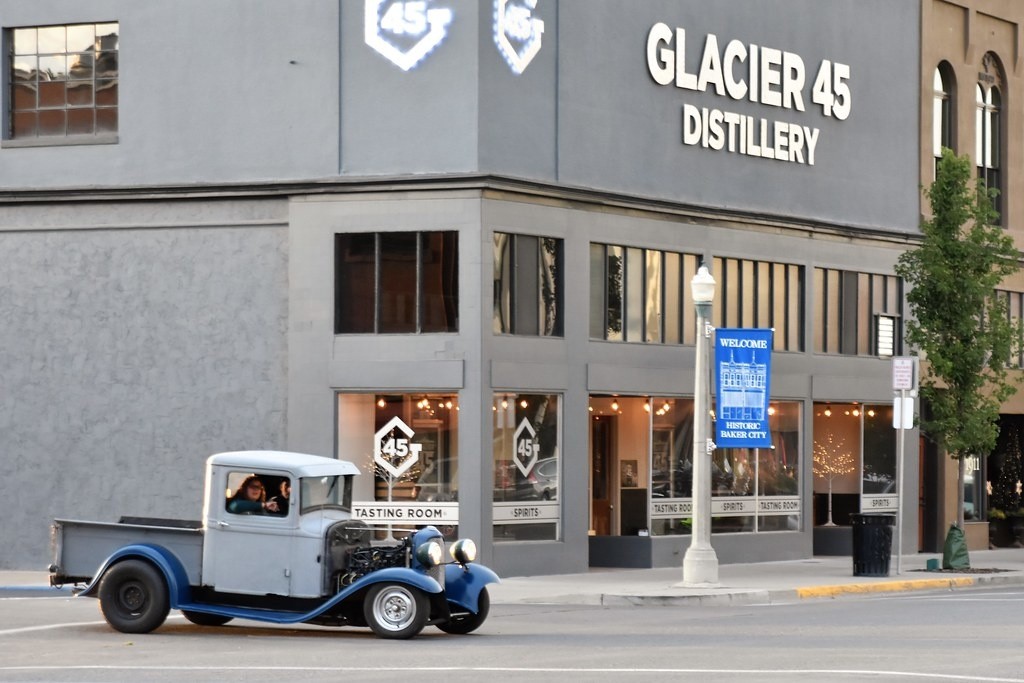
[48,448,501,641]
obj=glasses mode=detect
[248,483,263,490]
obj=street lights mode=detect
[680,265,722,584]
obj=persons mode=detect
[227,476,290,517]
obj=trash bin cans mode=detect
[849,512,896,577]
